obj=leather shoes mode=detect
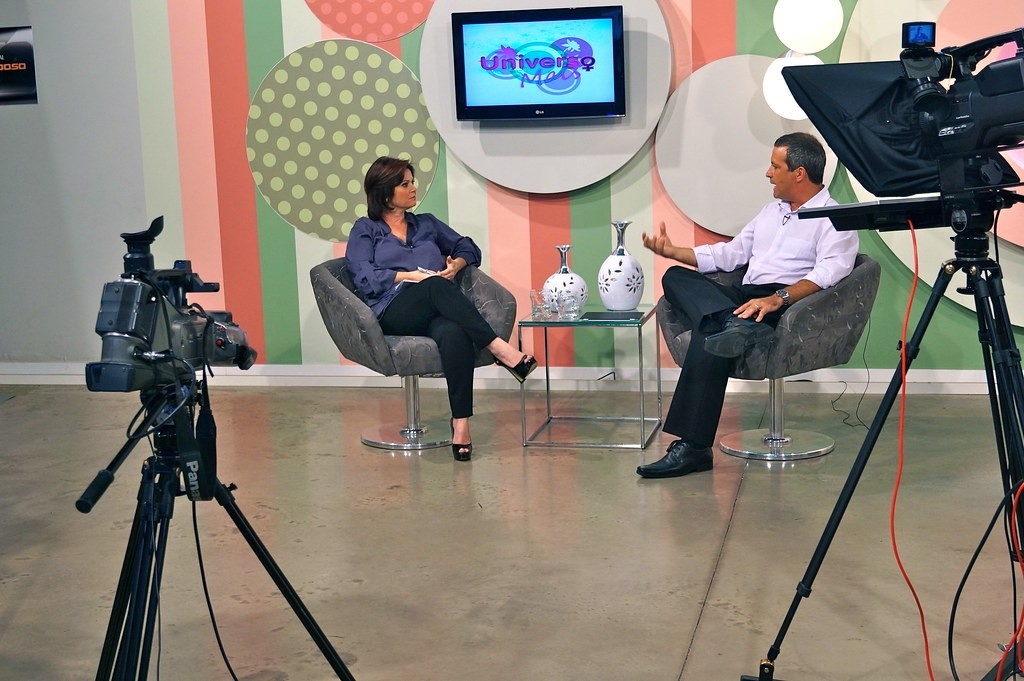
[637,441,713,477]
[703,317,773,358]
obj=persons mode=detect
[345,156,538,461]
[636,132,860,478]
[912,27,928,42]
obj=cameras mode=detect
[85,216,257,393]
[783,22,1024,193]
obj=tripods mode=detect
[72,396,356,680]
[742,192,1024,680]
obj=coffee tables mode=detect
[516,298,664,453]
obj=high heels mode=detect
[449,417,472,461]
[492,353,536,378]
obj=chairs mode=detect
[653,252,883,462]
[309,254,518,453]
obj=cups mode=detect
[527,287,554,319]
[556,295,580,320]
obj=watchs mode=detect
[776,290,790,305]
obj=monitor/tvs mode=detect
[450,6,628,120]
